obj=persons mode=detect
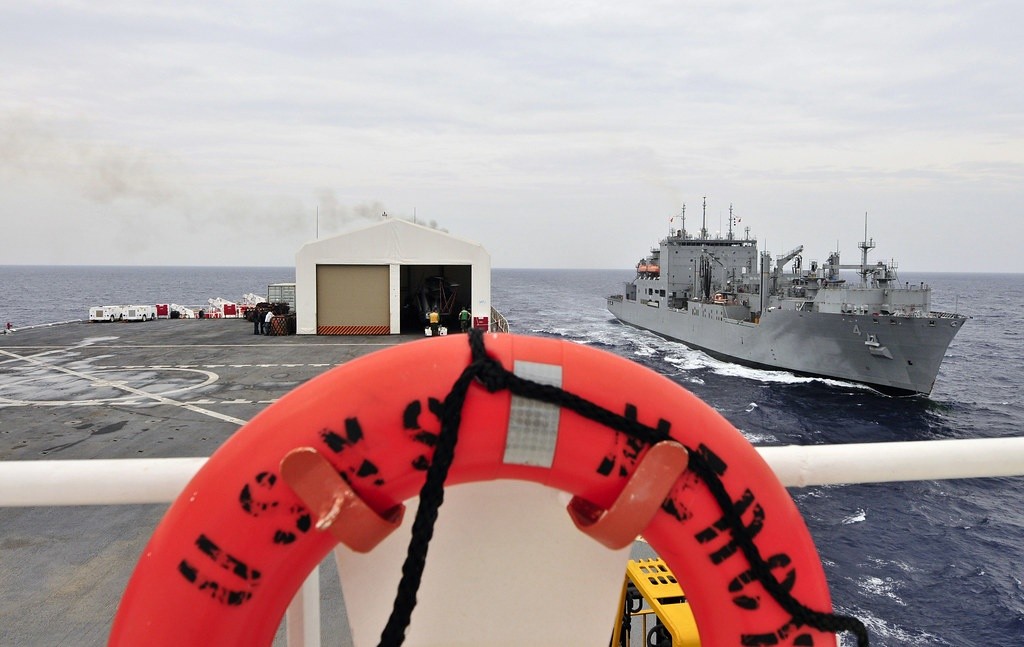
[430,308,439,336]
[459,307,470,333]
[253,308,274,336]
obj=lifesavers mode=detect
[108,334,837,646]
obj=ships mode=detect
[607,196,969,395]
